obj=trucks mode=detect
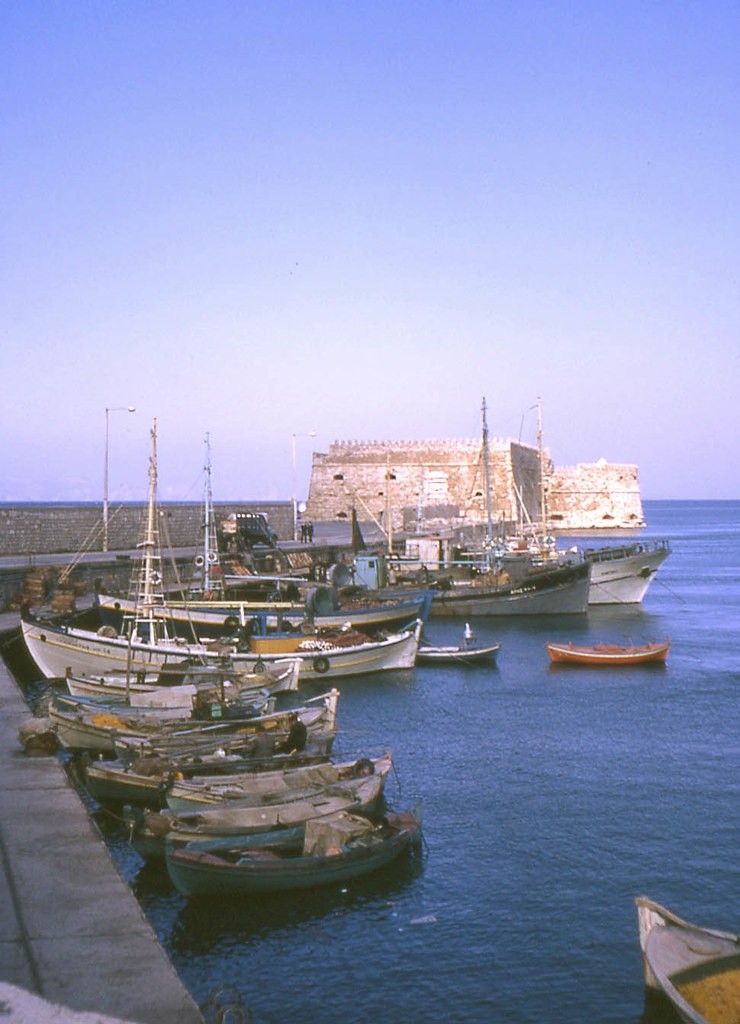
[201,510,280,552]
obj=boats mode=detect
[163,808,423,903]
[633,895,740,1023]
[546,633,671,668]
[17,392,671,891]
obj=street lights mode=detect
[102,406,137,552]
[292,432,318,542]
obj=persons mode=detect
[278,712,307,754]
[300,522,308,543]
[20,598,31,619]
[244,724,275,773]
[307,521,314,543]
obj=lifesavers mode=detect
[223,614,293,631]
[312,654,331,674]
[352,757,376,780]
